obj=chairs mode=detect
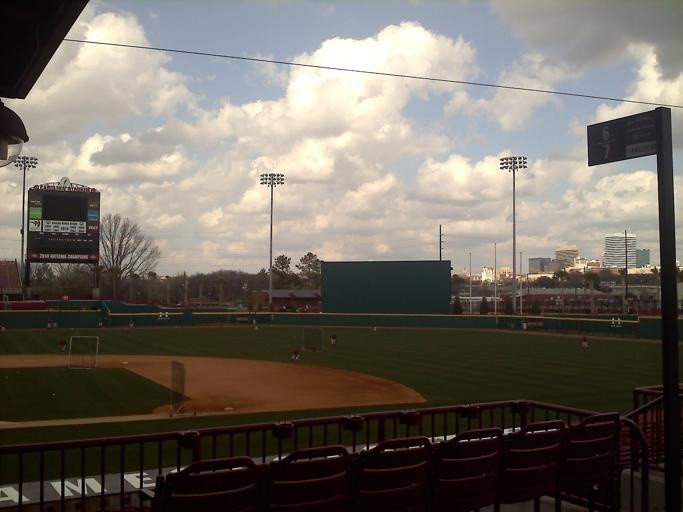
[138,410,619,512]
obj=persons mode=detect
[288,350,302,360]
[129,319,134,329]
[60,338,67,351]
[582,335,589,351]
[330,334,337,345]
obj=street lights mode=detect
[500,155,527,313]
[14,155,38,280]
[260,172,285,306]
